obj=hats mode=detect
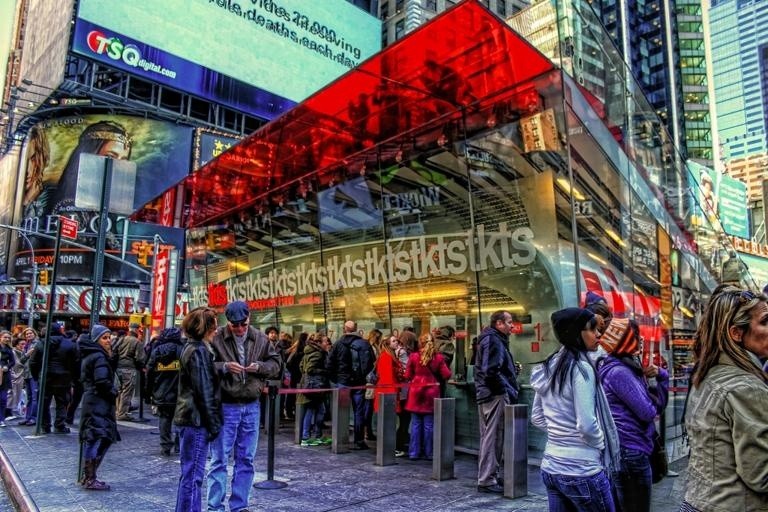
[91,325,111,342]
[598,317,641,357]
[551,307,595,343]
[129,323,140,328]
[225,301,249,324]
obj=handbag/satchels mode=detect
[350,340,371,374]
[366,371,378,385]
[302,378,323,395]
[651,437,669,485]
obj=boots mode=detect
[80,438,111,490]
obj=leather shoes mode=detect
[477,483,503,493]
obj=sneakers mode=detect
[301,437,332,446]
[395,450,404,456]
[54,427,70,433]
[18,420,36,426]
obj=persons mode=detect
[206,300,282,511]
[172,305,223,512]
[699,167,718,213]
[260,319,457,458]
[679,283,768,511]
[1,319,89,434]
[530,306,623,511]
[472,310,523,493]
[23,124,51,207]
[56,120,134,202]
[593,313,670,512]
[584,303,614,370]
[75,323,123,491]
[106,322,187,458]
[583,289,608,311]
[755,284,768,384]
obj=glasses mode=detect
[232,323,247,327]
[729,291,752,325]
